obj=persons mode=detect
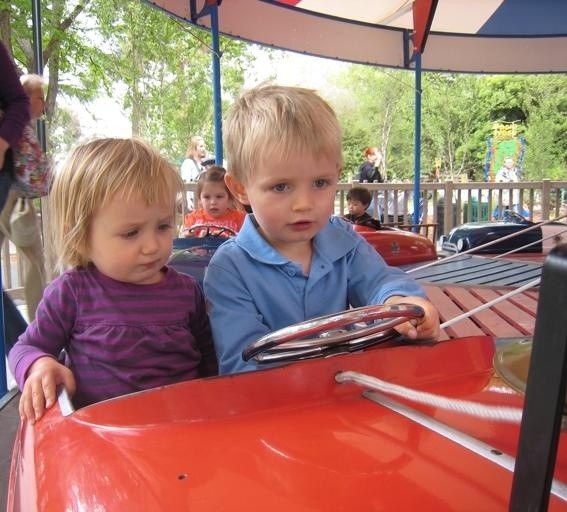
[202,85,440,377]
[0,38,29,354]
[435,166,439,182]
[445,167,449,175]
[356,148,382,216]
[178,166,246,239]
[494,155,521,206]
[466,167,475,181]
[19,73,48,119]
[180,135,208,211]
[8,137,218,426]
[341,186,380,230]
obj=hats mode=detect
[9,196,38,247]
